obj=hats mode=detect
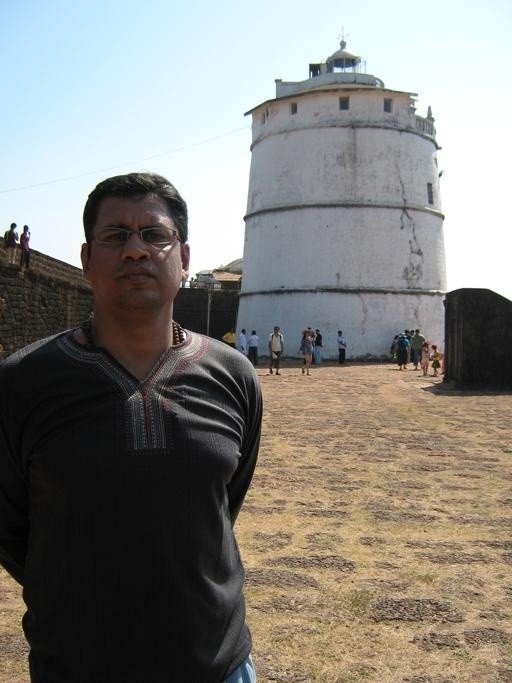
[399,333,407,338]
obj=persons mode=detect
[223,328,236,349]
[269,326,283,375]
[300,327,323,375]
[0,173,261,683]
[240,329,248,358]
[20,225,30,268]
[246,331,259,366]
[392,329,447,377]
[337,330,347,364]
[5,223,18,266]
[190,274,215,288]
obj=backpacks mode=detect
[399,343,408,354]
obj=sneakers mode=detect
[302,367,312,376]
[398,367,438,378]
[269,368,281,375]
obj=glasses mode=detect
[90,225,183,246]
